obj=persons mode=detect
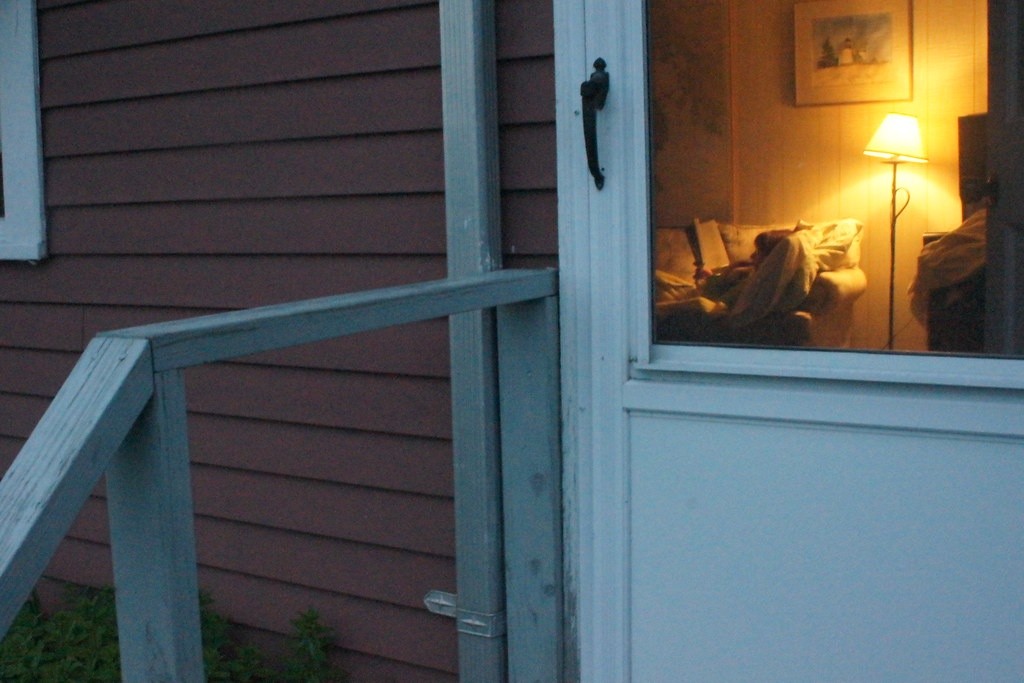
[688,231,796,310]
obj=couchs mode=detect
[652,220,869,351]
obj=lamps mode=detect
[861,114,928,353]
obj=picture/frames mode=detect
[793,0,914,108]
